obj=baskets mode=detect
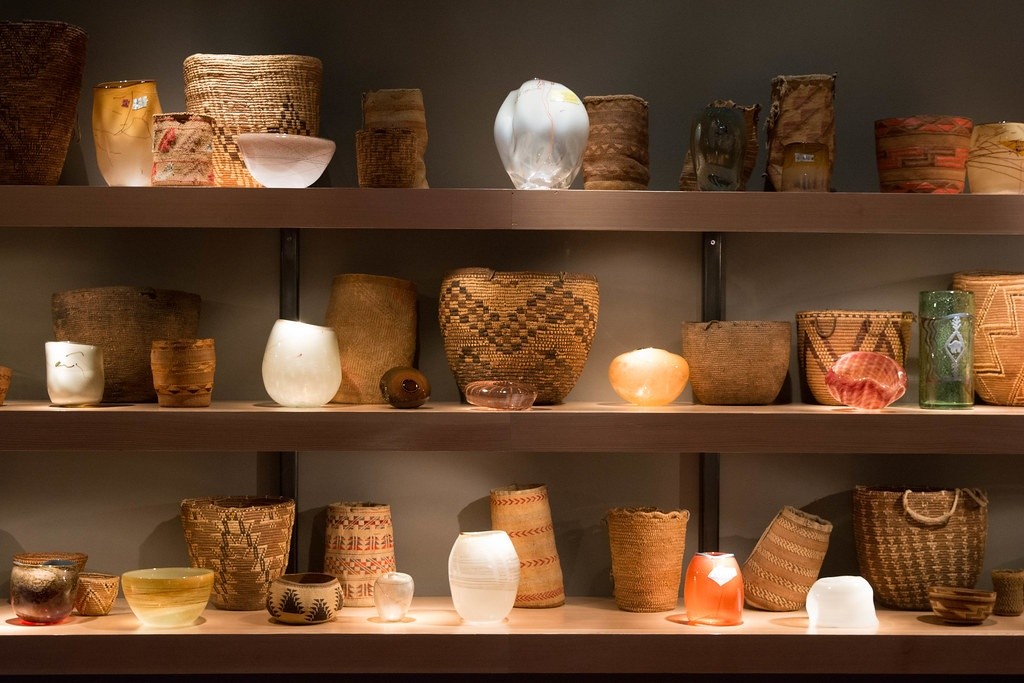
[794,308,913,408]
[324,273,419,405]
[851,482,989,609]
[1,19,90,189]
[990,566,1023,616]
[490,485,565,608]
[181,52,323,185]
[11,547,89,571]
[355,129,415,186]
[603,505,691,612]
[178,492,296,612]
[951,272,1024,406]
[324,501,397,609]
[74,573,119,617]
[439,266,600,407]
[682,319,792,407]
[740,494,837,610]
[49,277,202,403]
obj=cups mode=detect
[46,342,104,405]
[150,337,216,407]
[966,123,1024,193]
[917,290,975,410]
[262,320,343,406]
[783,143,830,193]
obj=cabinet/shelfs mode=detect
[0,50,1024,683]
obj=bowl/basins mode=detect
[875,116,973,193]
[121,567,215,629]
[233,134,336,188]
